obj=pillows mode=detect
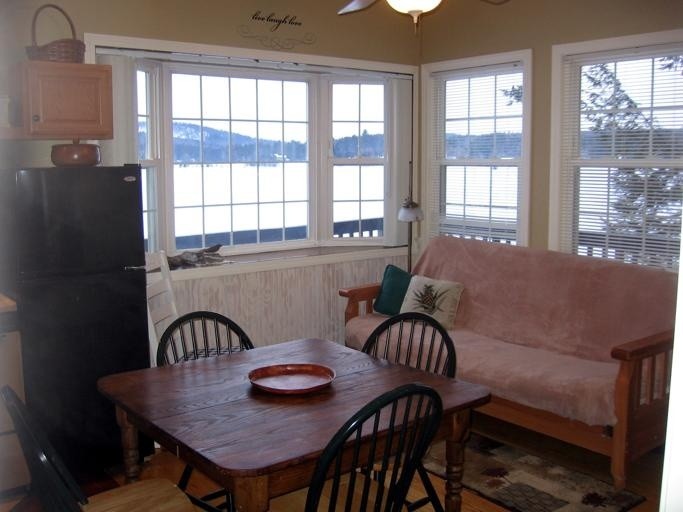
[373,263,463,330]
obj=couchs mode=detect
[337,234,678,488]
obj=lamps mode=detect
[385,0,444,33]
[396,162,425,272]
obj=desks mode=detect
[95,336,491,511]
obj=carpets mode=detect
[420,430,646,511]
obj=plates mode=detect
[247,362,337,397]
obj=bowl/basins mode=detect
[50,143,102,167]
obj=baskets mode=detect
[25,3,86,64]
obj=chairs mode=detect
[156,310,257,511]
[0,384,196,511]
[305,312,457,511]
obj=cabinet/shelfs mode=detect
[9,58,114,141]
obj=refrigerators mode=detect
[0,162,156,486]
